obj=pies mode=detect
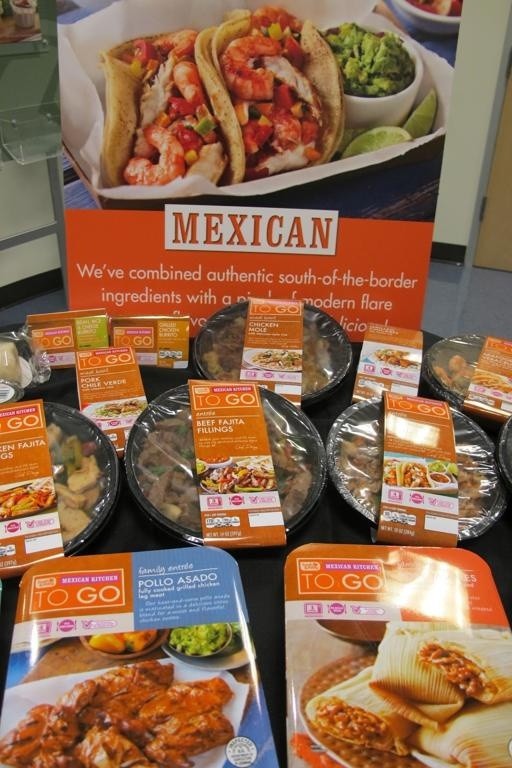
[102,1,345,194]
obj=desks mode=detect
[0,318,512,766]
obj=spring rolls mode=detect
[304,555,512,768]
